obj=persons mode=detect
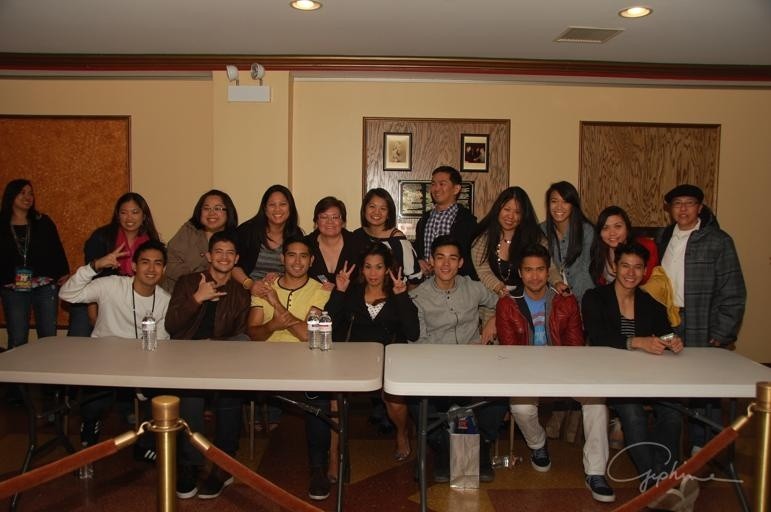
[652,181,747,457]
[61,166,681,501]
[0,178,70,407]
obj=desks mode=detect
[1,334,388,512]
[381,343,771,510]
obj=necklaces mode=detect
[131,282,155,339]
[10,220,36,269]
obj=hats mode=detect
[664,184,704,205]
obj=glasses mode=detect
[202,206,227,216]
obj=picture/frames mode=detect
[398,179,475,224]
[460,133,490,173]
[382,131,413,171]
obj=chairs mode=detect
[54,385,697,470]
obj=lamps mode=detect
[225,63,272,103]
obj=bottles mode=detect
[141,309,156,352]
[503,445,510,470]
[318,311,332,352]
[306,310,319,350]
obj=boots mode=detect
[433,438,450,483]
[480,440,495,483]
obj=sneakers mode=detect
[529,443,552,474]
[688,461,720,478]
[176,453,203,500]
[197,456,238,500]
[644,471,700,512]
[77,416,103,447]
[585,475,617,503]
[327,459,340,486]
[136,445,158,462]
[393,430,411,460]
[306,461,330,501]
